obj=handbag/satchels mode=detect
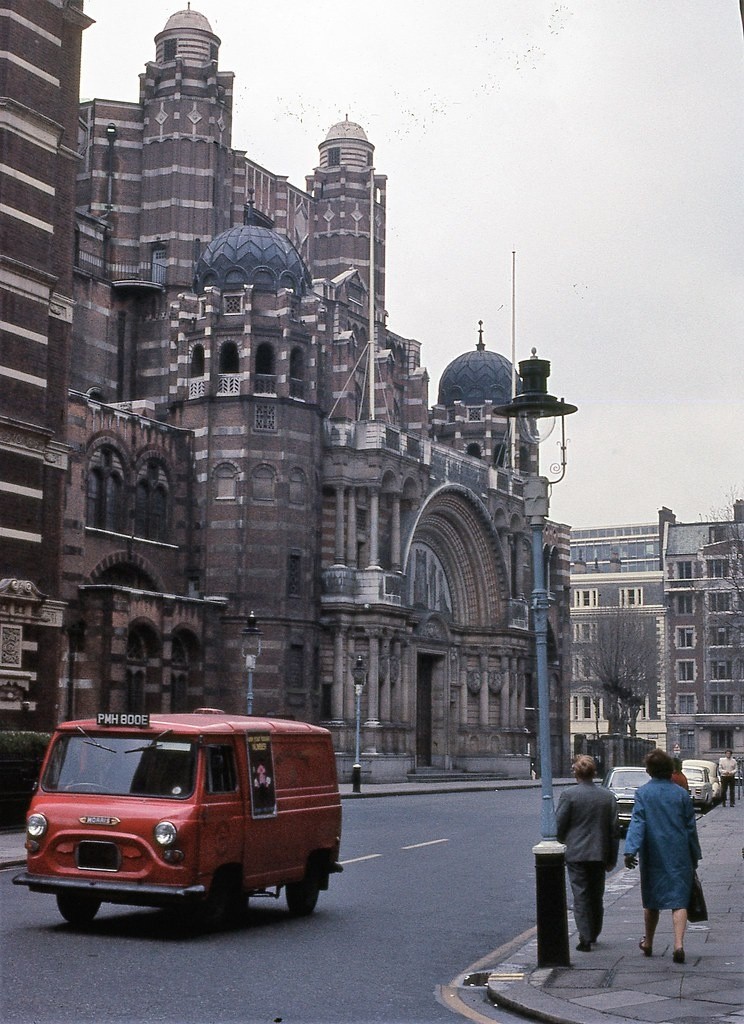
[687,871,708,923]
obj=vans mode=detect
[11,705,344,929]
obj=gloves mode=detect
[624,855,638,869]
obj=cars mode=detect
[681,765,714,814]
[599,767,653,829]
[683,759,722,807]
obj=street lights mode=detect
[352,655,368,792]
[241,610,264,715]
[491,346,577,969]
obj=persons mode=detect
[624,750,702,962]
[719,750,738,807]
[555,756,619,951]
[670,758,688,790]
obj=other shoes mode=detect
[730,804,735,807]
[576,937,596,952]
[722,803,726,807]
[672,947,685,963]
[639,940,652,956]
